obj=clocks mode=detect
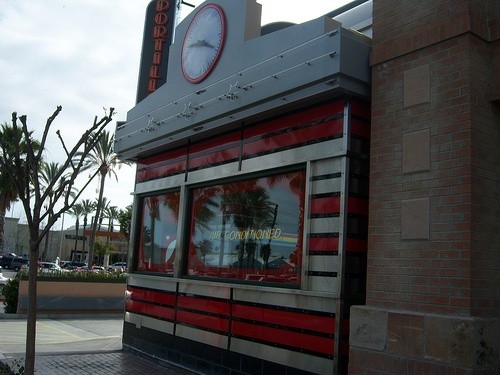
[180,3,227,83]
